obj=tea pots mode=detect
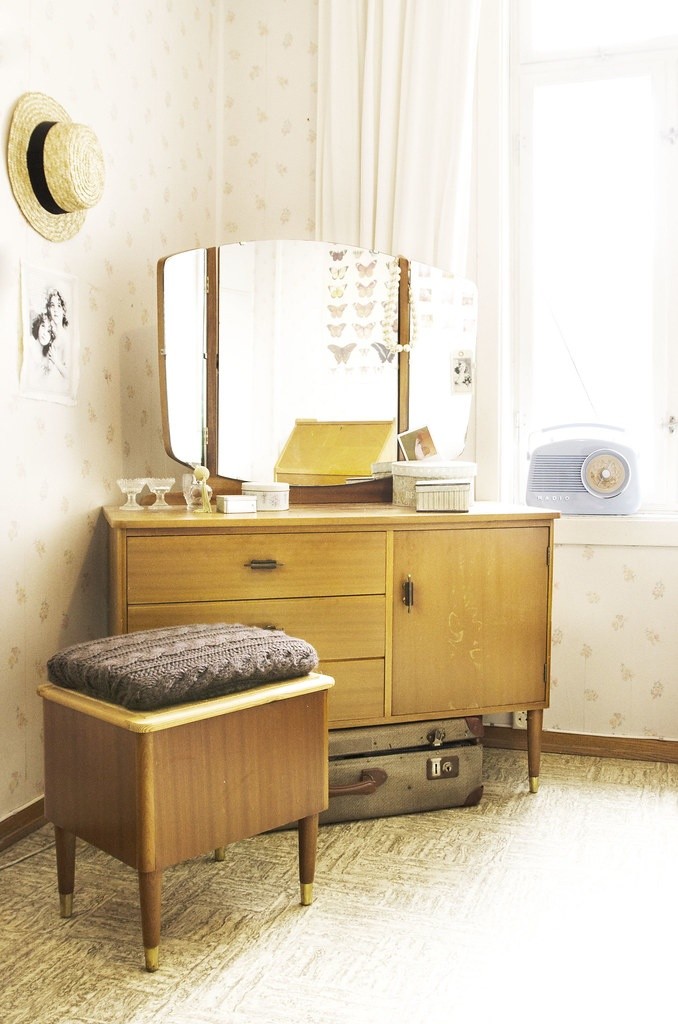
[183,474,212,510]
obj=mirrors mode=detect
[138,239,480,506]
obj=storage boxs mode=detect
[216,495,257,514]
[416,479,471,513]
[392,460,478,508]
[241,482,291,512]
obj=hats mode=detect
[8,93,106,242]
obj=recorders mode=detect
[526,423,642,514]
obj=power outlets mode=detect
[512,710,528,730]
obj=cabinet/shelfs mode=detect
[100,504,560,794]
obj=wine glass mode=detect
[146,478,176,509]
[116,478,146,510]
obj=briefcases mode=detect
[263,715,484,833]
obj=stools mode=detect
[36,621,336,973]
